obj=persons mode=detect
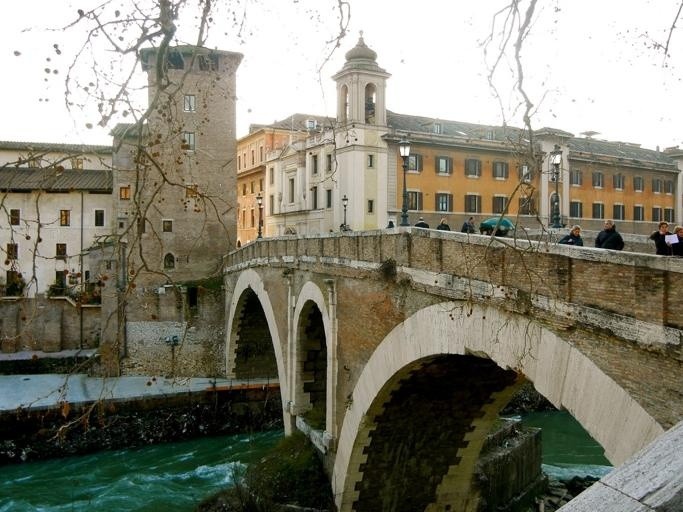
[595,219,624,250]
[672,225,683,257]
[559,224,583,246]
[650,221,672,255]
[387,221,394,228]
[415,216,429,228]
[461,216,476,233]
[437,218,450,230]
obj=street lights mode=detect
[398,134,412,227]
[341,194,349,231]
[255,192,265,239]
[549,143,563,229]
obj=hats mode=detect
[603,219,614,225]
